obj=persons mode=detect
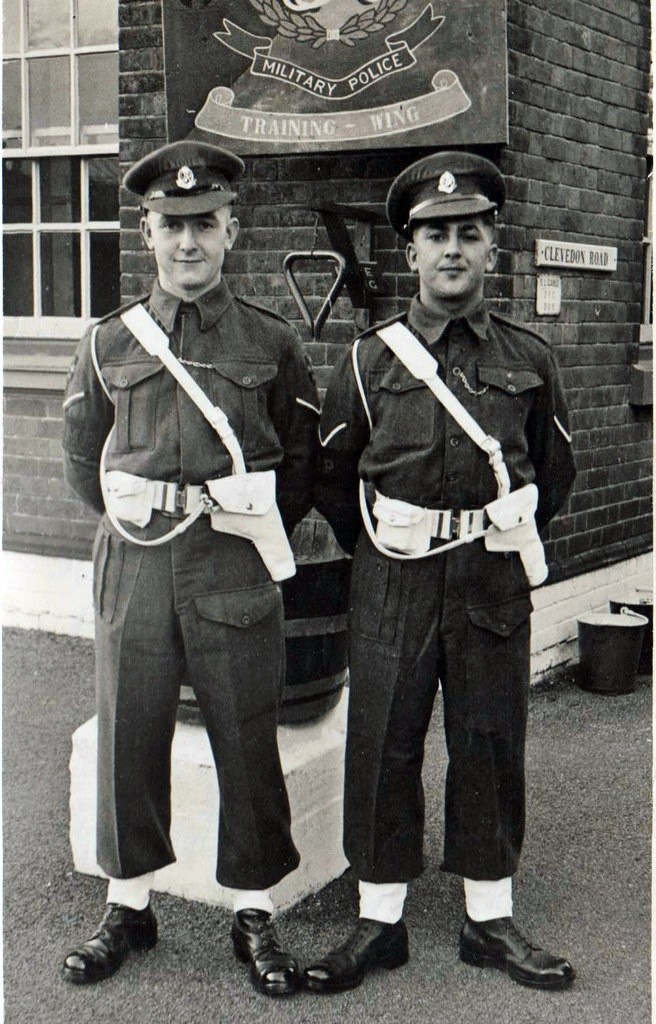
[302,151,577,994]
[58,140,323,998]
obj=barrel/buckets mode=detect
[170,519,352,727]
[609,592,653,676]
[577,613,648,695]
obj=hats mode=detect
[386,151,508,244]
[123,140,246,217]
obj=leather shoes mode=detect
[229,908,298,999]
[299,919,412,993]
[60,902,160,982]
[458,912,578,988]
